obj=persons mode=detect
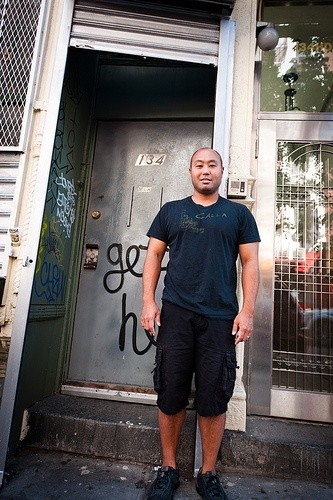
[140,149,262,500]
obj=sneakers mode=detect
[196,467,228,500]
[147,465,182,500]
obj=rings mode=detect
[144,320,149,322]
[247,335,249,339]
